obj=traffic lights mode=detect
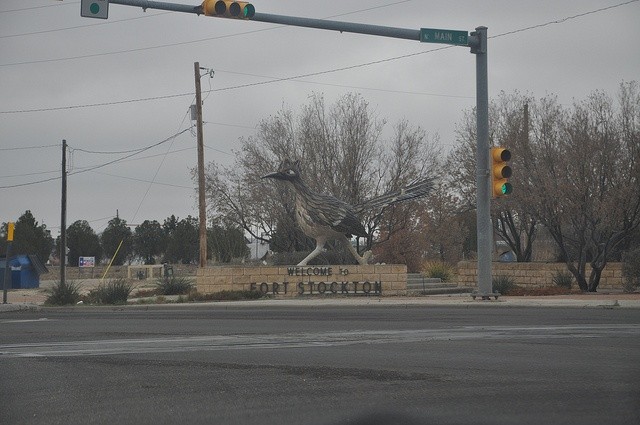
[491,146,513,199]
[202,0,256,21]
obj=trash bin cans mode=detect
[0,255,49,290]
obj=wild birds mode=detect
[261,158,442,266]
